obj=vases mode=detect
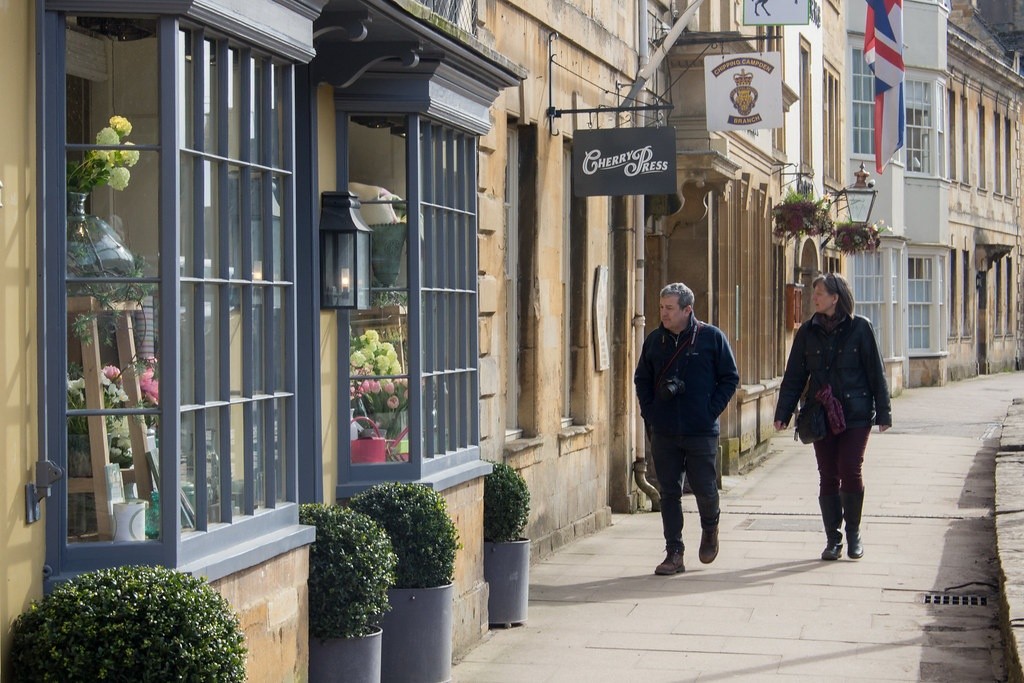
[67,190,136,296]
[66,434,116,535]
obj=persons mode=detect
[634,282,740,575]
[774,273,891,560]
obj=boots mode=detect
[839,486,864,559]
[699,515,719,563]
[819,495,844,560]
[655,545,685,574]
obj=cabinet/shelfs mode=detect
[66,296,152,541]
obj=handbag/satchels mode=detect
[794,389,847,444]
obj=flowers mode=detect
[67,115,140,295]
[67,353,160,470]
[349,330,409,441]
[771,184,836,248]
[833,213,894,259]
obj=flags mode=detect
[863,0,904,174]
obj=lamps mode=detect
[321,189,374,312]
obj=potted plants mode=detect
[299,503,399,683]
[480,458,531,627]
[348,480,465,683]
